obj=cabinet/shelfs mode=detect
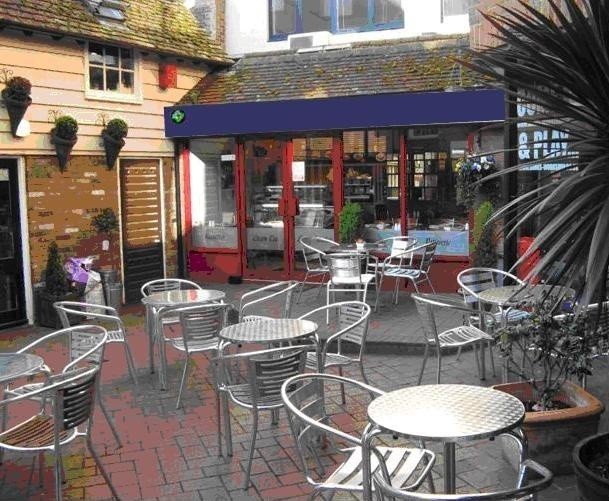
[249,158,389,230]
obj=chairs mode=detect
[211,343,325,491]
[321,253,381,326]
[141,278,235,410]
[1,324,123,488]
[323,236,418,308]
[272,300,376,434]
[456,267,609,390]
[373,240,439,313]
[281,373,435,501]
[296,235,340,304]
[234,280,300,384]
[53,301,141,408]
[0,364,123,501]
[410,291,495,385]
[372,458,554,501]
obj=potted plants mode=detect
[102,117,129,170]
[2,75,33,138]
[337,200,369,273]
[49,114,79,174]
[491,289,605,480]
[33,241,82,330]
[91,208,119,270]
[469,201,504,316]
[446,0,609,501]
